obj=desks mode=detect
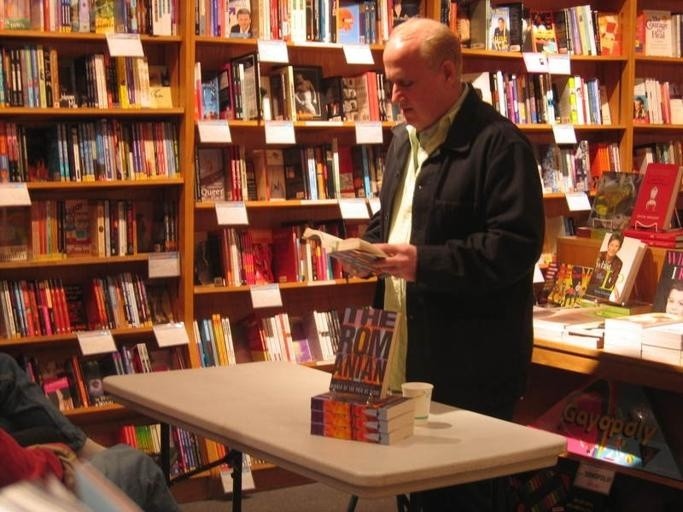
[100,361,568,512]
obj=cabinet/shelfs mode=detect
[190,0,630,501]
[630,0,683,230]
[0,1,211,504]
[531,235,682,511]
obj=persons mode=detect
[0,351,178,512]
[666,281,683,317]
[585,232,624,301]
[338,17,545,511]
[1,426,145,512]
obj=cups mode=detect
[400,381,434,421]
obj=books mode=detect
[300,225,391,279]
[603,312,683,358]
[540,260,593,309]
[194,1,425,45]
[634,138,683,193]
[630,163,683,230]
[566,321,604,349]
[196,218,373,288]
[583,232,649,307]
[464,71,613,125]
[532,141,621,194]
[579,297,652,319]
[634,8,683,58]
[440,0,623,56]
[0,274,179,341]
[1,1,180,39]
[587,171,644,233]
[1,42,179,109]
[16,338,188,412]
[532,306,599,345]
[114,419,271,477]
[622,227,683,249]
[194,50,406,122]
[641,320,682,367]
[310,391,415,445]
[0,190,180,263]
[634,76,682,125]
[653,250,683,317]
[194,311,342,368]
[331,308,401,400]
[195,139,392,202]
[0,115,182,184]
